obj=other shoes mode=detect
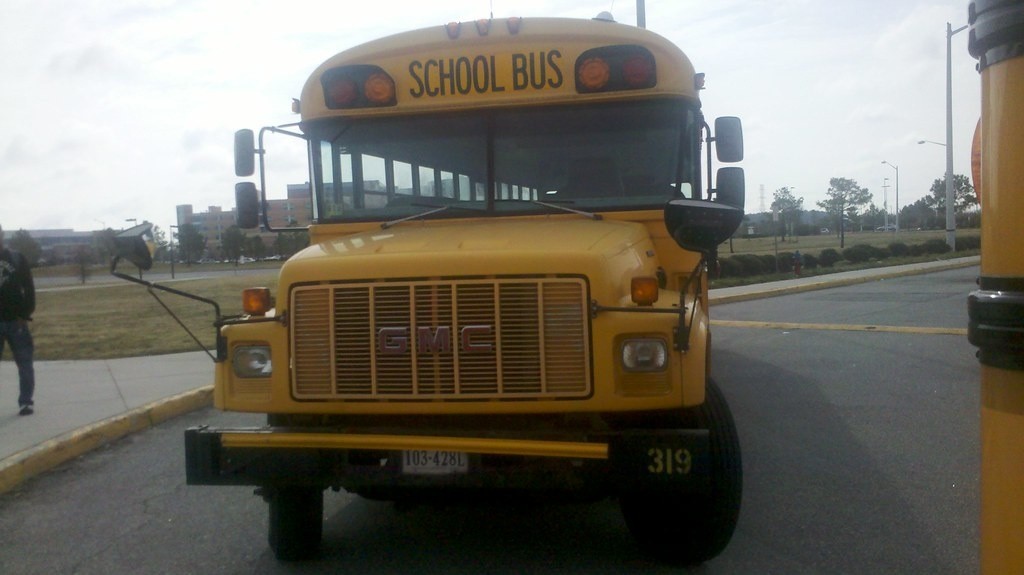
[19,405,34,415]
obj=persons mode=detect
[0,224,37,417]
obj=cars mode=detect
[878,224,897,231]
[821,227,829,232]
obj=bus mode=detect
[109,0,744,567]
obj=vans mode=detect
[243,258,255,263]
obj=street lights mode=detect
[880,159,899,233]
[126,219,143,280]
[883,177,889,232]
[917,139,955,253]
